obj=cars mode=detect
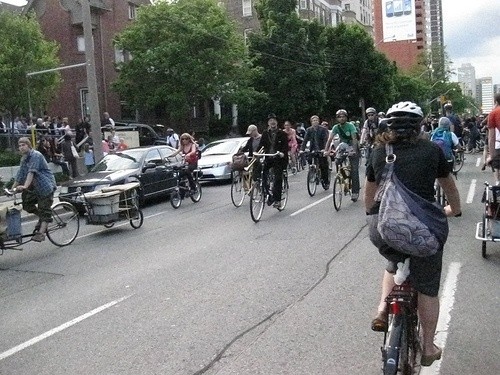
[196,138,251,182]
[58,146,202,213]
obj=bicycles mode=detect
[365,199,462,375]
[449,129,489,173]
[0,186,80,249]
[297,150,332,196]
[163,158,204,208]
[326,152,360,211]
[436,179,451,208]
[247,150,289,221]
[230,156,255,208]
[474,160,500,256]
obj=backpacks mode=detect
[431,127,454,160]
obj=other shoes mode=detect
[420,347,442,366]
[267,194,274,206]
[322,180,330,190]
[350,193,359,200]
[174,193,184,200]
[371,319,387,332]
[190,185,196,193]
[273,202,281,208]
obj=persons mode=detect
[166,129,179,149]
[421,104,488,189]
[364,102,461,366]
[233,107,386,207]
[0,112,95,181]
[3,137,57,242]
[166,132,198,200]
[488,93,500,187]
[101,112,128,157]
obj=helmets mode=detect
[366,107,377,114]
[336,109,348,116]
[385,101,423,128]
[444,104,452,109]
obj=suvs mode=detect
[59,121,160,171]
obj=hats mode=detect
[18,137,31,145]
[439,117,451,127]
[167,128,173,132]
[267,113,276,118]
[245,125,257,134]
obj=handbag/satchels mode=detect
[377,164,449,257]
[232,154,247,165]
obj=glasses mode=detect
[181,138,189,140]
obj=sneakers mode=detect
[31,233,46,242]
